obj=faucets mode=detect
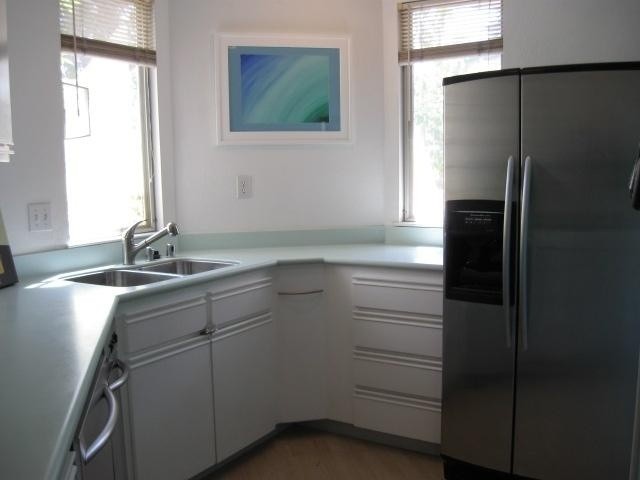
[121,218,179,266]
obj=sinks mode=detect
[59,265,180,288]
[125,254,240,277]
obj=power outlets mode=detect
[27,202,53,232]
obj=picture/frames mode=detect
[212,32,353,147]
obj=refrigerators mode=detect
[439,62,639,479]
